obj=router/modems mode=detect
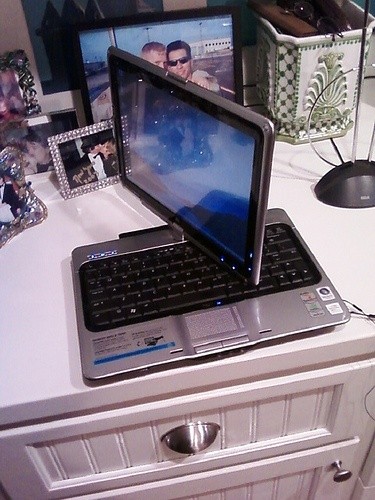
[307,0,375,208]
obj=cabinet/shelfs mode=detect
[0,337,375,500]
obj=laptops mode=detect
[71,45,352,380]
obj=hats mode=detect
[80,134,106,149]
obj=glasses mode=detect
[167,56,193,66]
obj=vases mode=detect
[252,1,375,145]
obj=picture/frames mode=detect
[1,49,40,123]
[69,9,243,128]
[0,148,48,247]
[46,121,120,200]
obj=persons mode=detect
[139,41,167,70]
[77,135,118,182]
[167,40,222,98]
[0,165,26,232]
